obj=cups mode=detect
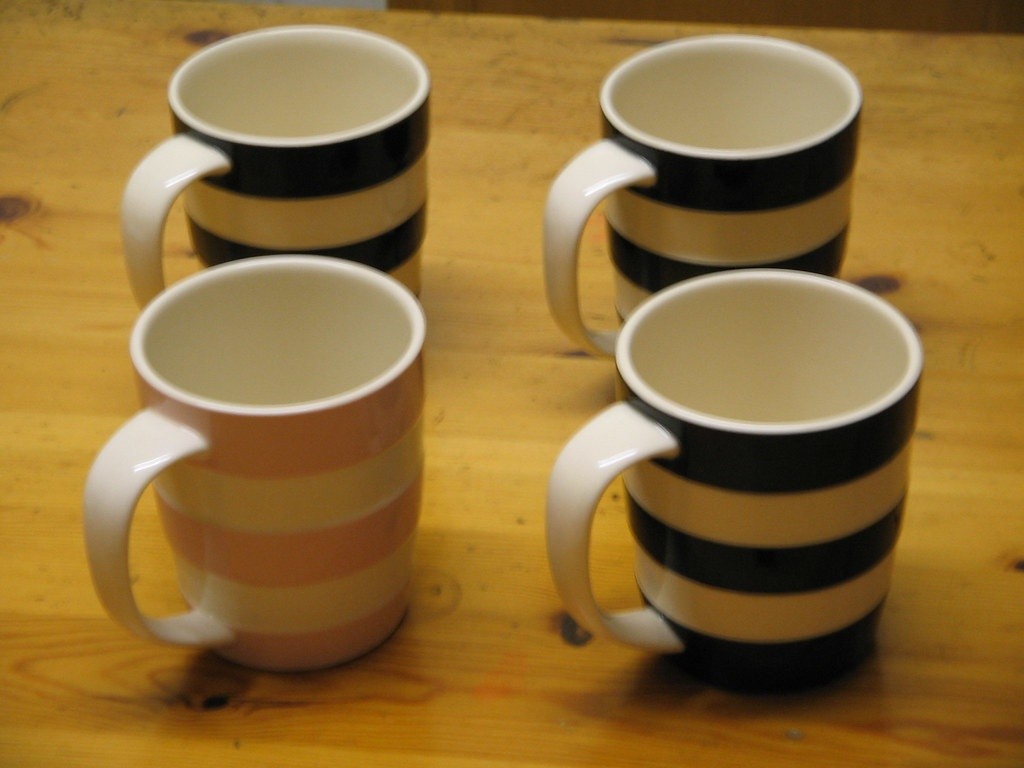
[545,267,926,701]
[539,32,863,361]
[119,23,431,310]
[82,254,428,674]
